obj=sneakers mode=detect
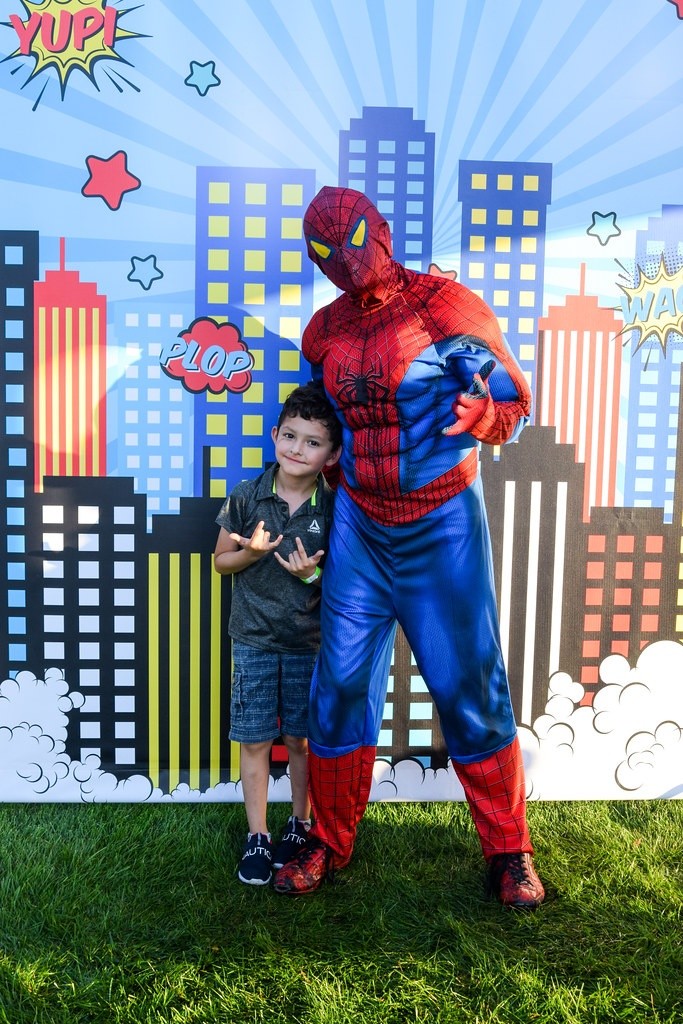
[273,816,309,869]
[273,837,351,895]
[485,852,545,910]
[237,833,273,885]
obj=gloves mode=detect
[441,360,496,437]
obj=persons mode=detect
[275,184,545,907]
[214,378,342,886]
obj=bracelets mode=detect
[300,566,321,584]
